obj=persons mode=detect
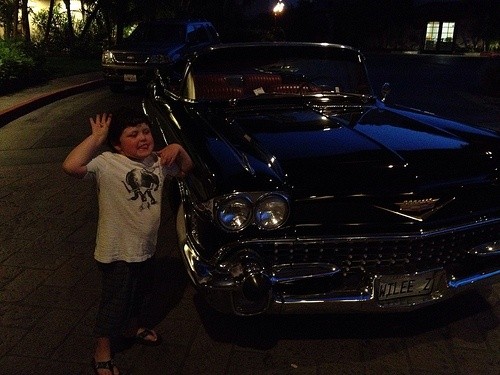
[63,108,193,375]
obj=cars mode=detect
[136,43,500,320]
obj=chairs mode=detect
[263,84,320,95]
[197,87,244,100]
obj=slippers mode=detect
[123,329,161,347]
[92,358,119,375]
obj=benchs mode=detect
[194,73,285,96]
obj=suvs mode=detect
[101,19,225,94]
